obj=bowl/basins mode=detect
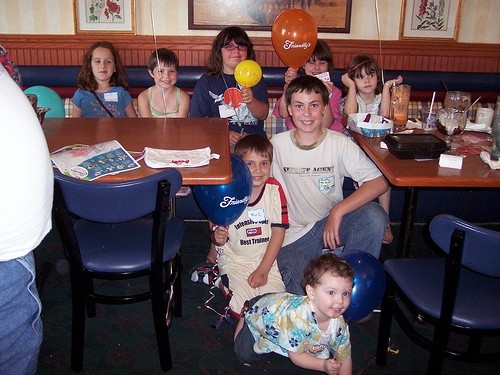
[382,134,451,160]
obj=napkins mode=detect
[479,150,500,170]
[407,117,432,131]
[464,117,492,135]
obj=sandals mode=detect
[188,263,218,287]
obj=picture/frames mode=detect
[397,0,467,43]
[187,0,352,35]
[72,0,137,36]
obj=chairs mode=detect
[375,213,500,375]
[48,165,188,375]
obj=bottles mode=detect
[491,95,500,160]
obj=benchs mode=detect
[13,59,500,231]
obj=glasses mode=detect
[223,44,248,51]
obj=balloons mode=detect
[24,86,65,118]
[272,9,317,70]
[194,153,251,228]
[339,249,386,323]
[234,60,262,89]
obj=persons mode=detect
[190,135,290,330]
[0,62,53,375]
[138,48,191,196]
[273,39,345,134]
[187,26,276,262]
[342,55,402,243]
[71,41,137,118]
[233,253,352,375]
[269,76,388,296]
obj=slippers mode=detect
[381,224,393,244]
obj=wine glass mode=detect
[437,108,467,155]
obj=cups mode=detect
[444,91,470,110]
[420,108,437,131]
[389,84,410,125]
[475,107,493,128]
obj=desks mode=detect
[340,113,500,259]
[40,114,234,314]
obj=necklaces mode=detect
[221,72,241,126]
